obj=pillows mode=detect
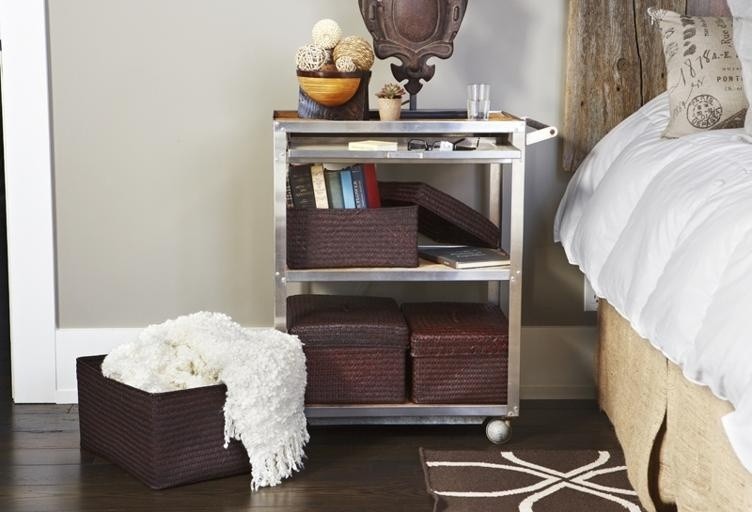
[646,6,745,136]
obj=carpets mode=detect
[419,439,643,512]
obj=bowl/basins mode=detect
[298,69,362,107]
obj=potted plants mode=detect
[375,81,405,121]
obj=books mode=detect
[287,161,381,211]
[418,245,510,270]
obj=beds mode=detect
[560,2,752,510]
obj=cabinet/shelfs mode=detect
[272,108,559,444]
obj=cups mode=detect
[466,84,491,121]
